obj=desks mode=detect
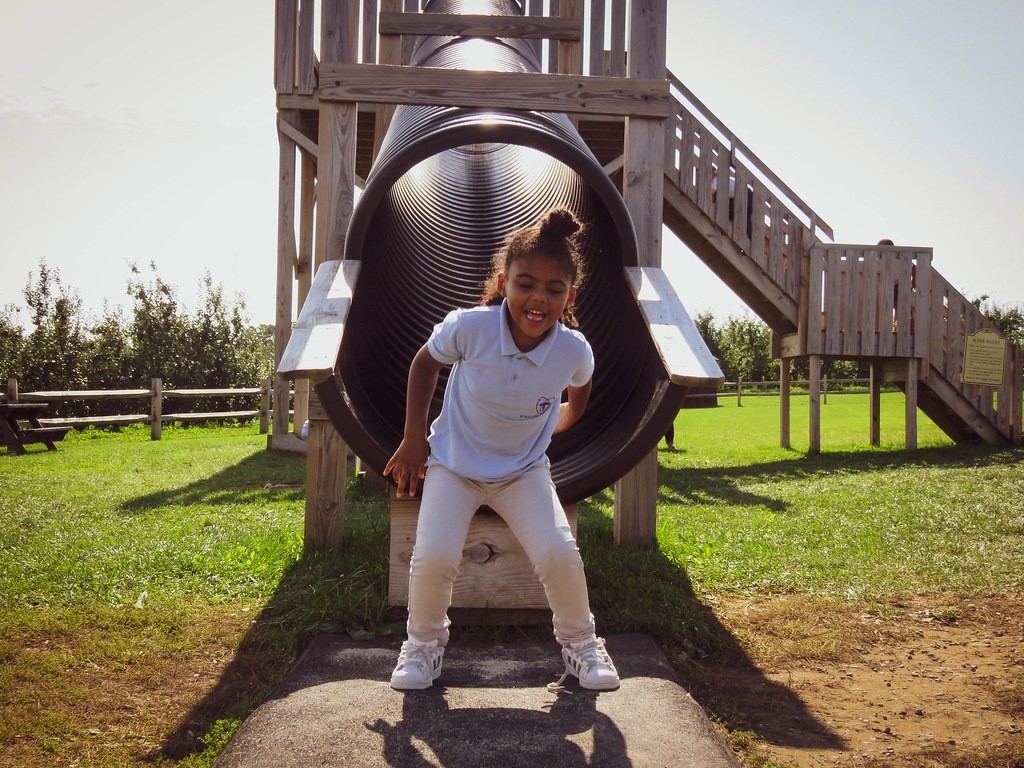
[0,403,59,455]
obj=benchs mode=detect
[0,426,73,455]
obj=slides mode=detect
[312,2,691,516]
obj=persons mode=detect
[383,208,621,690]
[665,422,675,449]
[877,239,917,334]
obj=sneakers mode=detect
[390,640,445,690]
[561,637,620,690]
